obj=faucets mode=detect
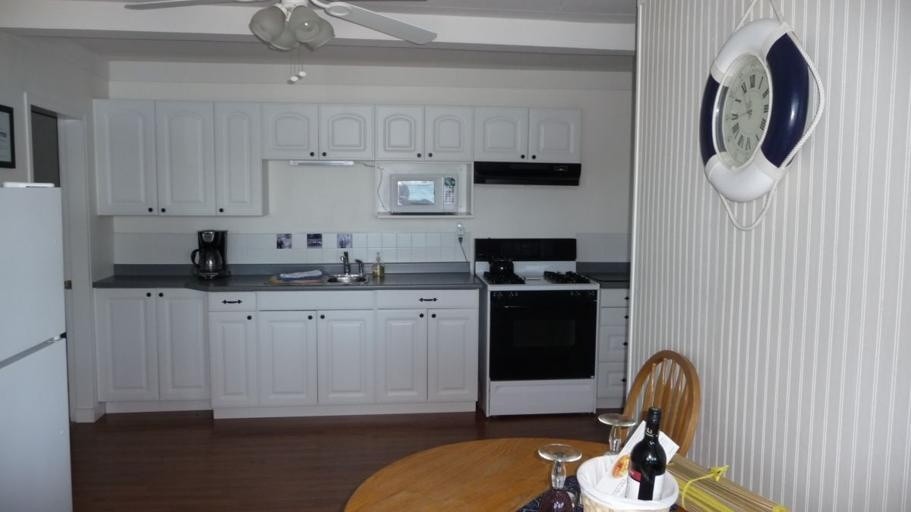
[340,251,351,273]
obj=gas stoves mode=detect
[481,270,597,303]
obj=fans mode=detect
[125,0,438,44]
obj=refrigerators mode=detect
[0,188,75,512]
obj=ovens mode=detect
[489,300,597,417]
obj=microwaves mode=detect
[387,173,458,215]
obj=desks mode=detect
[344,437,688,512]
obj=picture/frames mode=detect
[0,104,17,168]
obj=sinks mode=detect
[327,273,368,285]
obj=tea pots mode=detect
[485,248,515,278]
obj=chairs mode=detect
[621,349,701,457]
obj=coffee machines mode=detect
[188,227,230,282]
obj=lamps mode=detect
[248,0,335,52]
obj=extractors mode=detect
[475,161,582,186]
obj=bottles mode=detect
[622,405,668,502]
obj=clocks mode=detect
[698,18,809,204]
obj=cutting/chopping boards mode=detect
[268,276,322,284]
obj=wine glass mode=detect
[534,442,582,511]
[595,413,635,458]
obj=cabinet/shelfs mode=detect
[474,107,581,164]
[94,288,212,414]
[264,102,374,163]
[256,290,377,418]
[597,289,631,409]
[374,104,473,162]
[214,100,267,216]
[208,291,258,420]
[92,99,216,216]
[376,289,479,415]
